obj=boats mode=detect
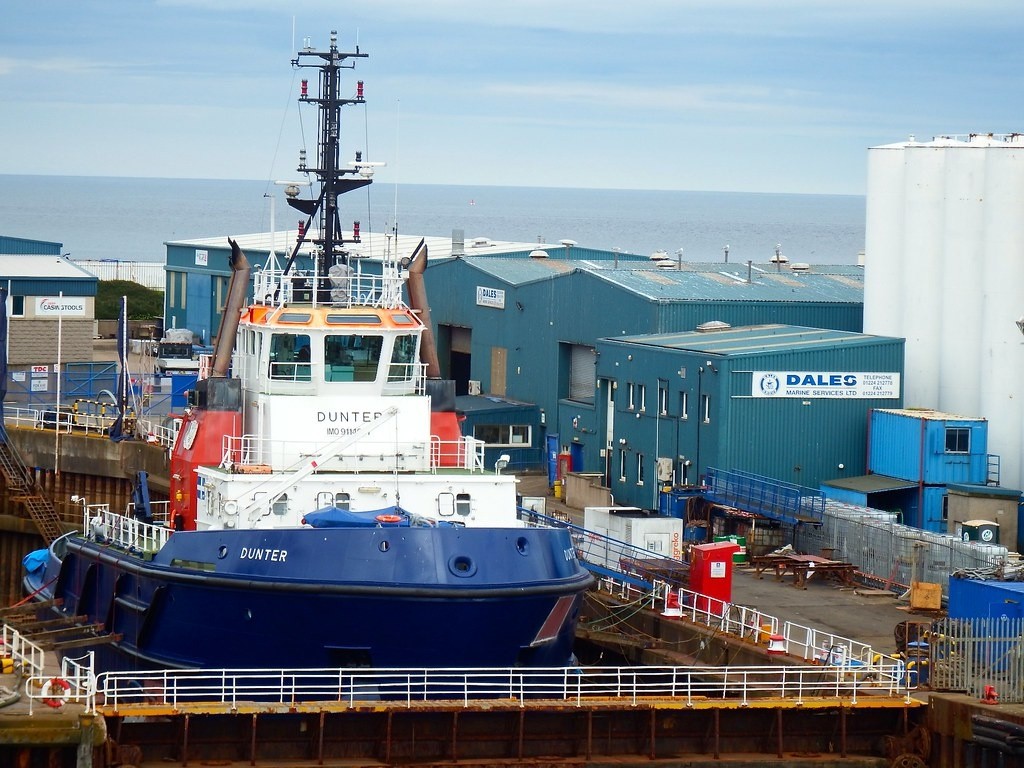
[17,29,596,704]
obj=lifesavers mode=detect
[41,678,72,708]
[201,356,210,378]
[375,515,402,522]
[169,509,176,535]
